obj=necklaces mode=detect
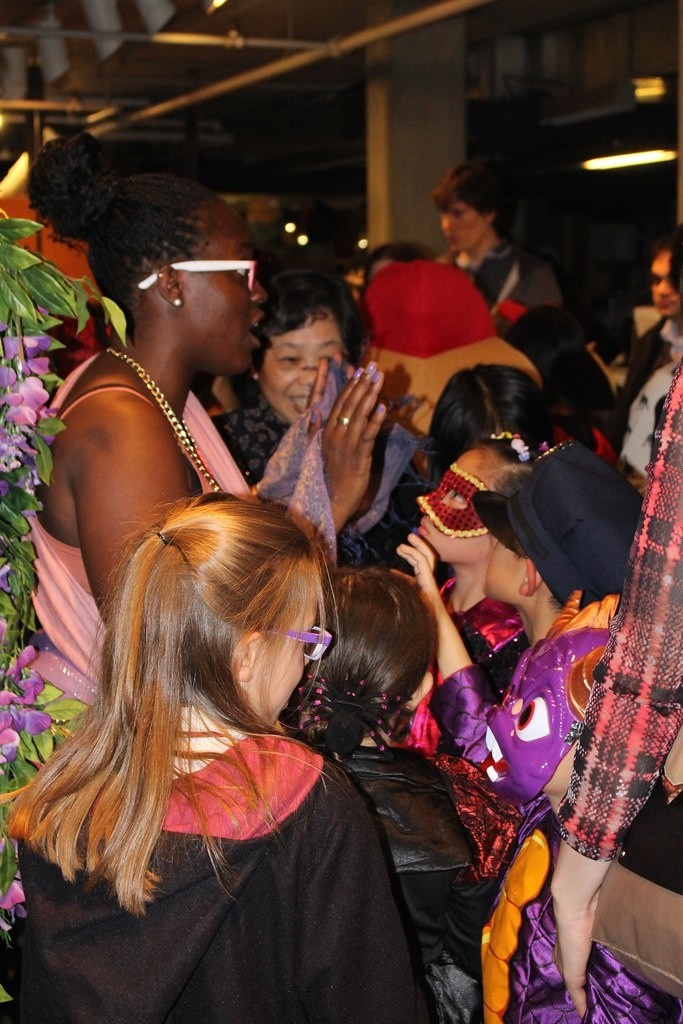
[104,346,222,494]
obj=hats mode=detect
[472,441,647,614]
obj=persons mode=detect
[0,118,683,1024]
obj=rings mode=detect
[337,414,348,426]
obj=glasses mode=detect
[138,260,260,293]
[247,623,333,662]
